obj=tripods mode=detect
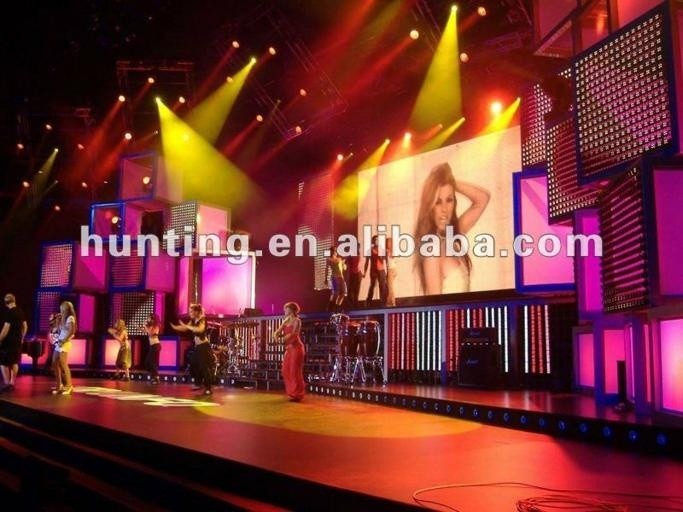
[330,323,386,385]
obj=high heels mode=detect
[52,384,65,395]
[62,385,74,395]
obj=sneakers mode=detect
[3,384,15,392]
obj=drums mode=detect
[213,336,236,360]
[340,320,380,357]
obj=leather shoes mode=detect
[110,374,120,380]
[151,376,156,382]
[120,376,130,381]
[204,389,212,395]
[152,378,160,385]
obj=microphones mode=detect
[48,313,62,326]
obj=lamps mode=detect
[111,216,122,227]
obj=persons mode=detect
[410,162,492,297]
[107,317,132,382]
[380,237,399,307]
[273,302,307,402]
[169,302,215,395]
[47,312,69,389]
[0,292,30,391]
[138,312,163,385]
[361,235,389,306]
[50,301,80,397]
[344,241,365,310]
[324,246,350,314]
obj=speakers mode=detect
[458,327,503,388]
[141,211,164,242]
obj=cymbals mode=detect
[228,323,259,327]
[207,322,222,327]
